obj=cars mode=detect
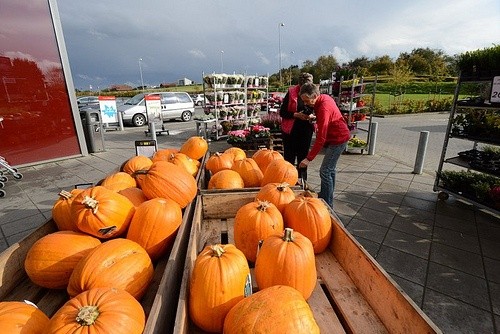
[76,95,99,105]
[79,104,100,122]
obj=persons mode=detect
[278,73,316,182]
[299,83,351,209]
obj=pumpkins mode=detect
[0,300,49,334]
[207,147,299,189]
[25,137,207,300]
[43,287,145,334]
[189,182,332,334]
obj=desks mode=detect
[196,119,218,140]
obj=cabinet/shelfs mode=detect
[203,76,269,139]
[316,82,376,151]
[433,71,500,221]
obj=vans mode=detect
[117,91,195,127]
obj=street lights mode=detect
[278,22,285,83]
[217,50,225,74]
[136,56,144,90]
[290,51,295,86]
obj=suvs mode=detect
[192,93,209,106]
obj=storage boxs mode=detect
[0,151,444,334]
